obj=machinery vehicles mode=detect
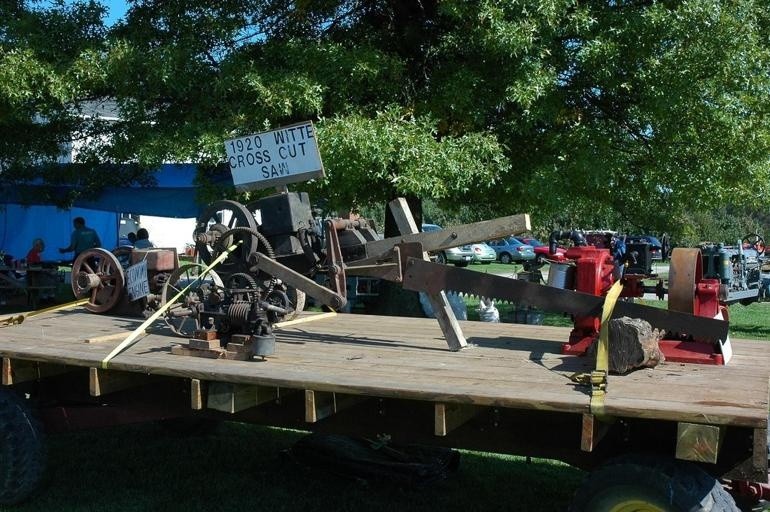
[685,231,770,309]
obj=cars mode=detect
[119,217,147,269]
[418,222,664,272]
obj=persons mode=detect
[26,239,45,262]
[128,232,137,245]
[58,217,101,266]
[129,229,153,263]
